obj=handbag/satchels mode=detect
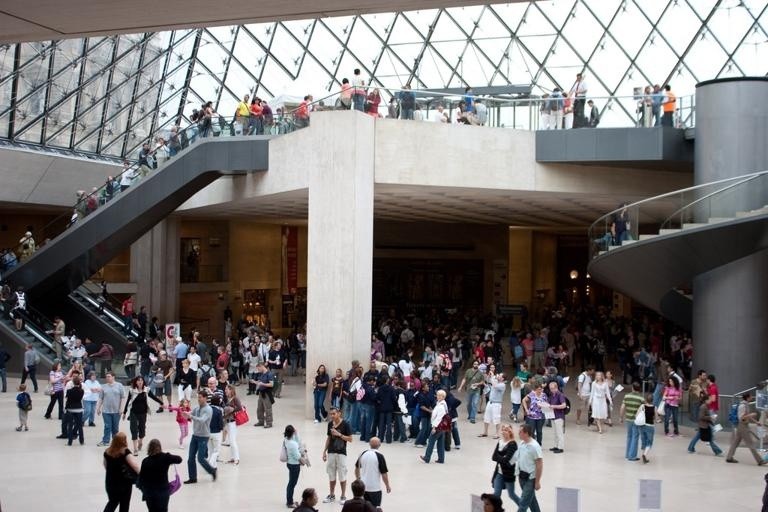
[44,381,55,395]
[280,446,287,461]
[588,405,594,426]
[699,426,712,441]
[634,411,646,426]
[435,413,451,431]
[235,410,249,425]
[153,371,165,388]
[657,400,666,416]
[169,475,181,494]
[349,390,357,403]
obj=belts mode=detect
[226,419,234,423]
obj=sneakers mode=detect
[435,461,444,463]
[455,445,460,448]
[642,455,649,464]
[254,422,264,426]
[97,441,109,446]
[156,407,163,413]
[625,457,640,462]
[212,468,217,479]
[168,405,172,412]
[413,444,424,447]
[726,458,738,463]
[550,447,563,452]
[420,455,429,464]
[340,496,347,504]
[322,494,335,503]
[183,479,196,483]
[666,432,673,436]
[717,450,725,456]
[56,433,68,438]
[758,461,767,465]
[265,424,272,428]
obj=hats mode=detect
[481,493,503,506]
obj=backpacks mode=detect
[729,400,747,424]
[21,393,33,410]
[200,366,212,386]
[550,393,570,415]
[575,374,586,390]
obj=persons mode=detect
[280,424,309,509]
[341,69,423,121]
[490,422,520,508]
[292,488,319,512]
[589,201,635,253]
[102,431,140,511]
[355,436,391,509]
[437,87,488,127]
[0,283,307,484]
[186,93,325,138]
[540,71,599,129]
[319,407,354,506]
[342,480,384,512]
[135,438,182,512]
[0,119,190,287]
[313,287,768,467]
[481,492,504,512]
[509,423,544,512]
[642,84,676,129]
